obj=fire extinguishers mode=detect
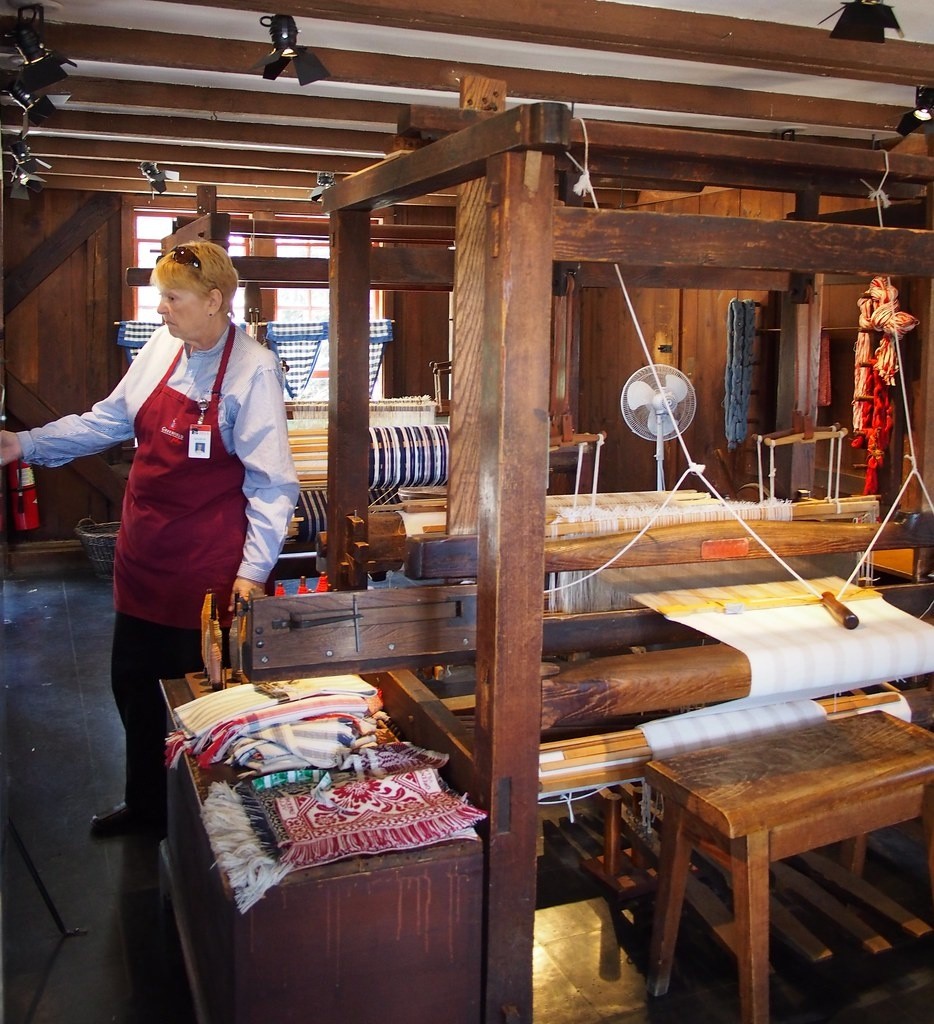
[8,457,39,531]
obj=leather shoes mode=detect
[90,801,163,835]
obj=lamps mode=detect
[249,13,331,86]
[817,0,904,44]
[888,83,934,137]
[139,162,180,200]
[310,173,336,203]
[0,0,76,199]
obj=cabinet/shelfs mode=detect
[158,674,484,1024]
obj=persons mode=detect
[0,241,299,833]
[196,444,203,452]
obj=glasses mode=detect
[156,245,202,272]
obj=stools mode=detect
[645,709,934,1024]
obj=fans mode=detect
[620,364,696,490]
